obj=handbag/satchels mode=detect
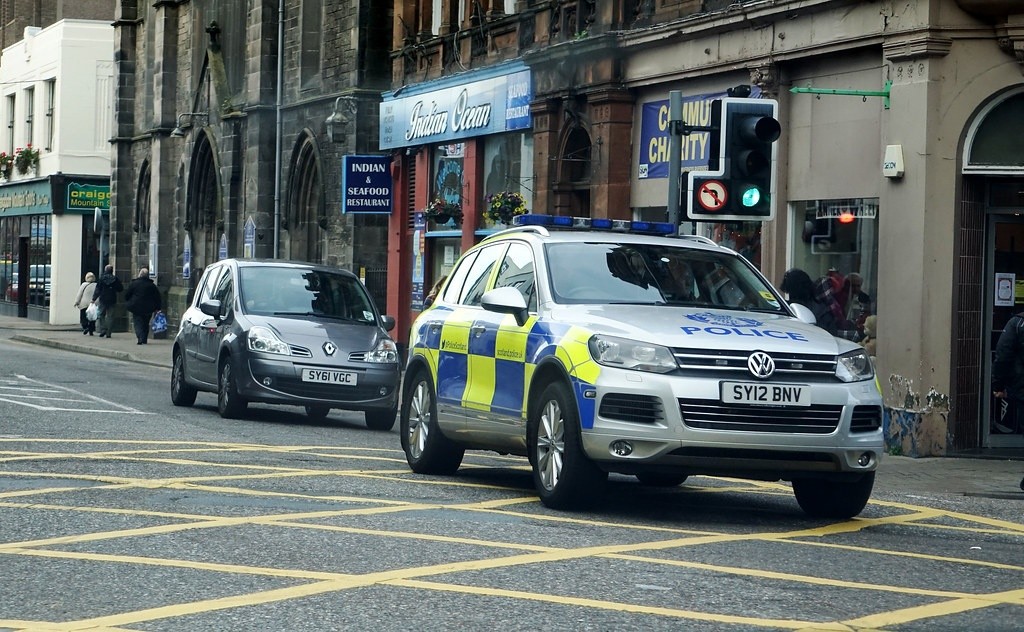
[86,302,97,321]
[151,311,167,333]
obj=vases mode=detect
[434,215,451,224]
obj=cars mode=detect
[6,265,51,305]
[171,258,401,432]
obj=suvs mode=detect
[397,214,885,517]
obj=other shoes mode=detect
[137,340,142,345]
[100,330,106,337]
[89,331,93,336]
[84,328,89,335]
[142,338,147,344]
[106,332,111,338]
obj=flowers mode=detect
[421,196,465,226]
[15,143,39,175]
[482,192,530,226]
[0,152,16,181]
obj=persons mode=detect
[125,267,164,345]
[74,265,122,338]
[781,264,878,356]
[990,313,1023,493]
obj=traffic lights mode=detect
[681,98,780,222]
[804,204,858,243]
